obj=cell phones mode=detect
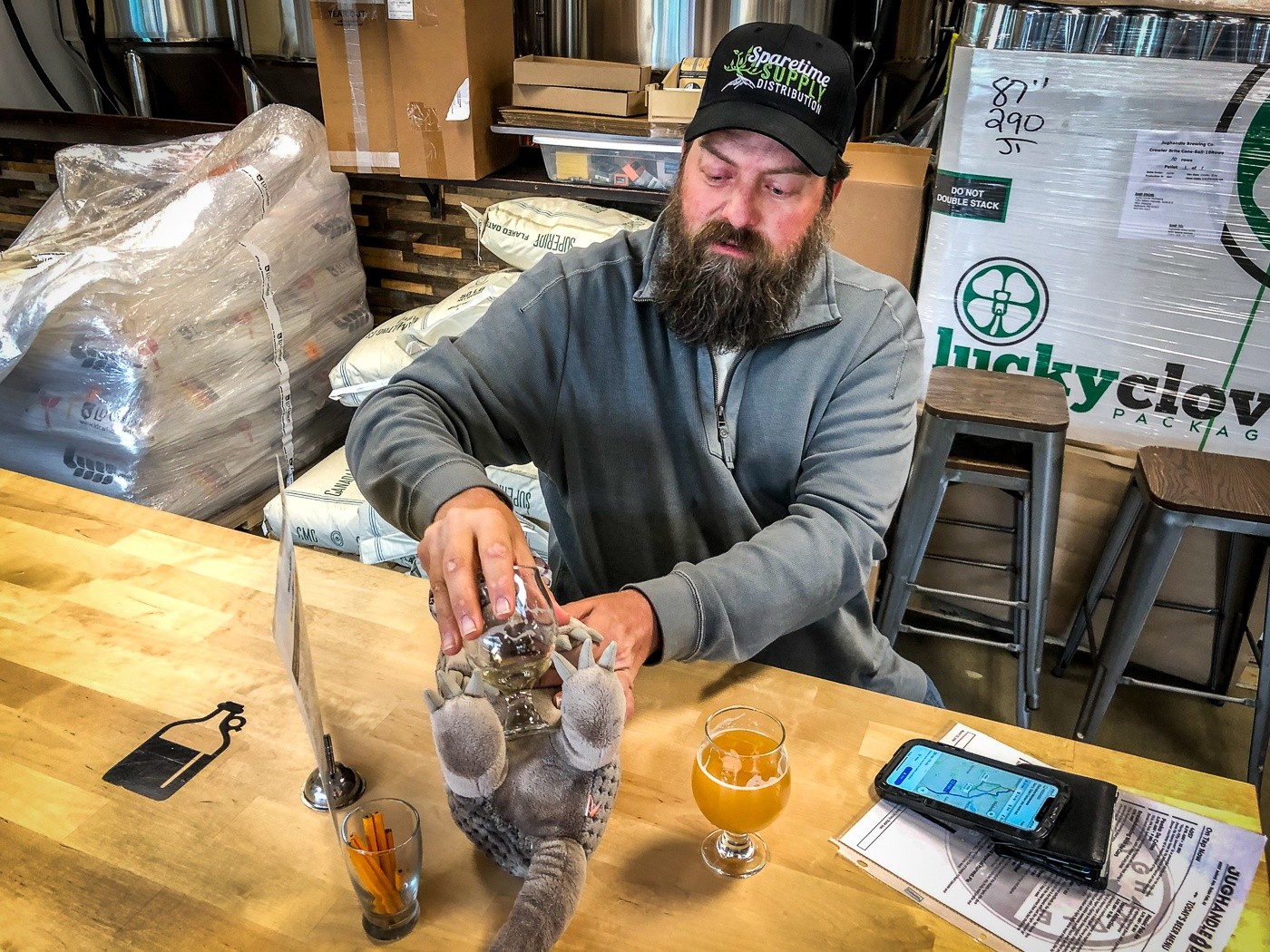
[874,739,1073,850]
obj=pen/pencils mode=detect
[384,829,396,872]
[347,832,405,915]
[396,869,405,892]
[363,814,380,871]
[373,811,394,881]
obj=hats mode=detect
[684,23,857,177]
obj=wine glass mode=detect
[463,566,560,741]
[691,705,791,879]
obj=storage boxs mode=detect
[385,0,514,180]
[309,0,399,174]
[513,54,649,92]
[532,136,683,193]
[825,143,934,291]
[511,84,647,117]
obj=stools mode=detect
[880,365,1270,783]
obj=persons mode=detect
[345,22,946,712]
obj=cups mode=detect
[341,798,423,943]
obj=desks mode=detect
[0,477,1270,952]
